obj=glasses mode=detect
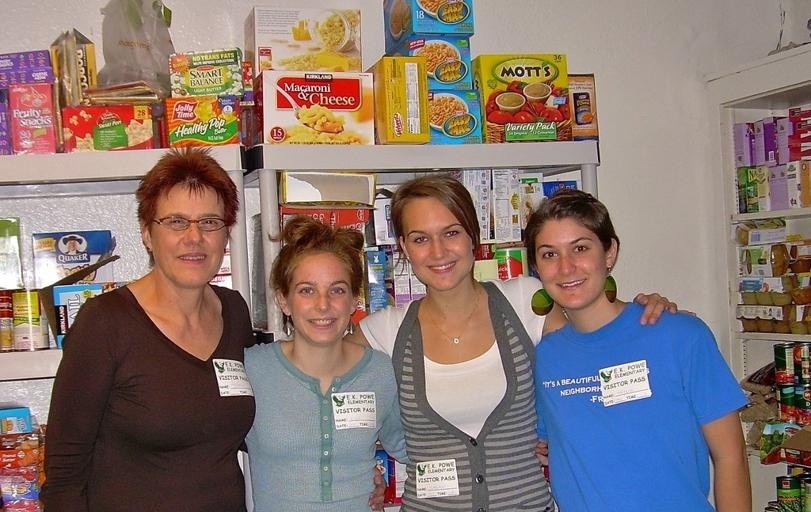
[153,216,226,231]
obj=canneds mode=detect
[12,291,50,350]
[773,343,811,427]
[776,472,811,512]
[0,288,12,353]
[497,247,529,282]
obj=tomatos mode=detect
[486,81,570,126]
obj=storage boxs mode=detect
[0,0,602,510]
[728,98,811,216]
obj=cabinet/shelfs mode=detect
[704,37,810,512]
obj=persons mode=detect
[39,143,387,512]
[342,174,698,511]
[523,189,752,512]
[243,216,549,511]
